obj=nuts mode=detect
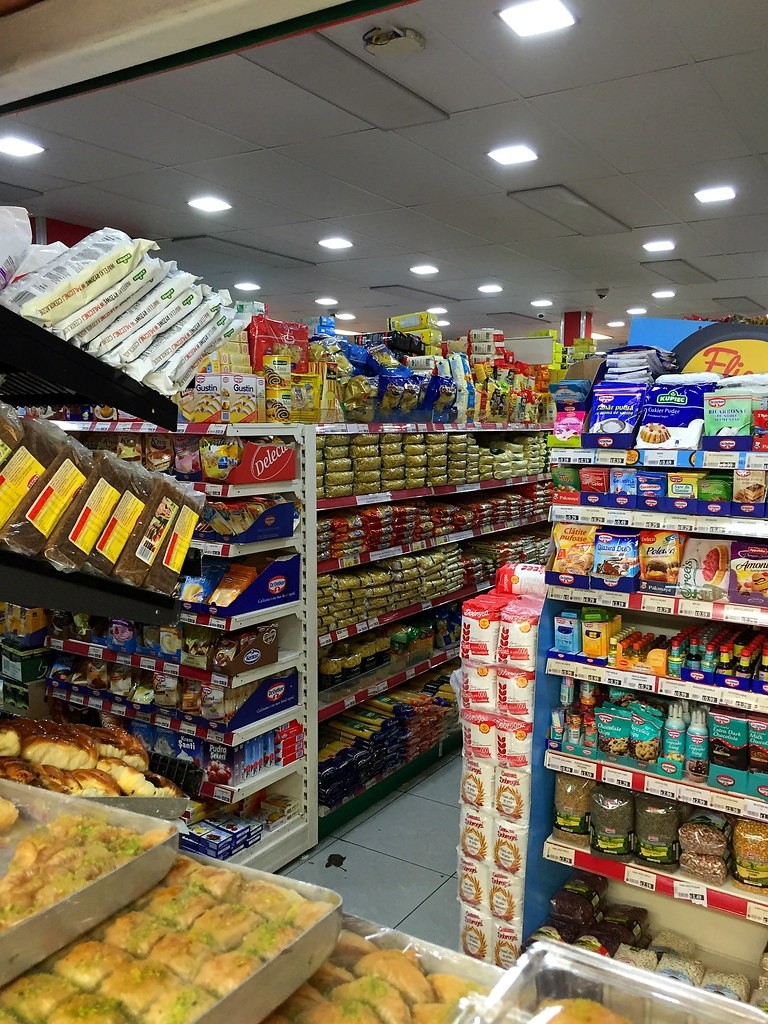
[679,824,727,887]
[615,932,749,1002]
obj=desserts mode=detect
[649,570,664,580]
[603,558,622,576]
[559,554,593,574]
[640,422,670,443]
[701,545,728,584]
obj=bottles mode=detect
[608,625,667,667]
[667,624,768,682]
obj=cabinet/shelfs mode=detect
[42,417,319,873]
[313,421,554,840]
[0,305,183,629]
[517,447,768,958]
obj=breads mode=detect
[0,713,629,1023]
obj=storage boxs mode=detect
[0,611,305,862]
[213,501,296,542]
[221,353,250,366]
[0,778,181,987]
[221,342,249,352]
[192,530,215,541]
[173,469,201,482]
[201,442,296,485]
[179,849,344,1024]
[543,343,768,801]
[354,311,516,377]
[194,374,223,423]
[223,374,267,423]
[236,301,265,316]
[221,365,253,374]
[147,466,175,477]
[182,556,236,613]
[230,331,248,342]
[197,348,220,374]
[204,552,301,617]
[471,935,768,1024]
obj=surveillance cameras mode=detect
[596,289,608,299]
[328,309,337,317]
[538,313,545,319]
